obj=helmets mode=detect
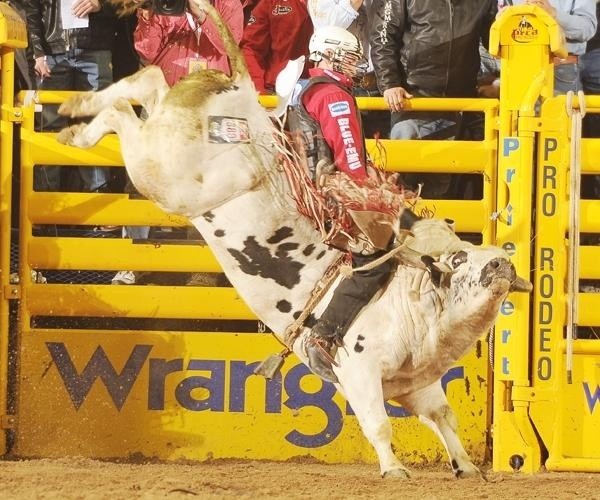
[307,26,365,64]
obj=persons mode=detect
[1,1,600,287]
[287,25,421,384]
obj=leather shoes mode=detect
[301,327,339,387]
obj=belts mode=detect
[551,53,582,67]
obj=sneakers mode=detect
[111,266,153,284]
[186,272,220,287]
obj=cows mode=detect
[57,0,534,482]
